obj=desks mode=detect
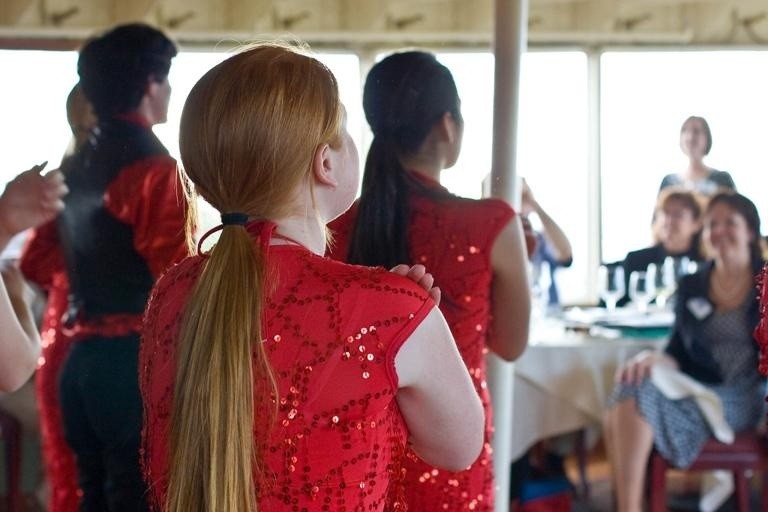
[488,307,695,511]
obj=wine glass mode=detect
[626,268,657,314]
[645,259,678,308]
[595,263,626,313]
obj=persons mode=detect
[19,78,100,512]
[51,19,200,512]
[0,163,69,407]
[620,186,710,308]
[657,112,737,192]
[132,31,490,511]
[480,176,582,503]
[325,46,536,510]
[602,192,766,511]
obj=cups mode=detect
[663,254,690,285]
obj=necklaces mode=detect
[708,270,748,298]
